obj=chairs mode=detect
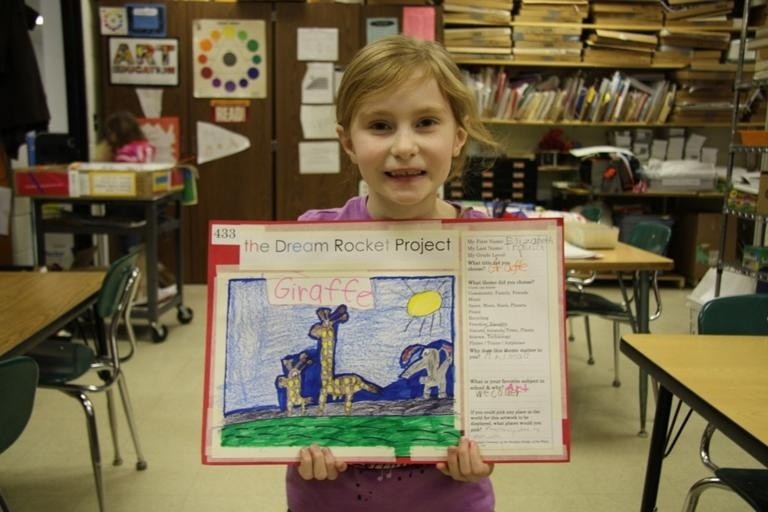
[0,246,147,512]
[677,294,768,512]
[565,205,672,388]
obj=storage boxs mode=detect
[13,164,186,200]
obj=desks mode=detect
[1,270,124,512]
[619,332,768,512]
[564,242,676,437]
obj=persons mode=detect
[283,33,497,512]
[79,111,155,251]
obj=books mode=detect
[737,25,767,112]
[438,0,735,64]
[462,68,738,125]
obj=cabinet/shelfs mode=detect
[32,178,195,345]
[432,0,768,298]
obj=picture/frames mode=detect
[107,36,181,88]
